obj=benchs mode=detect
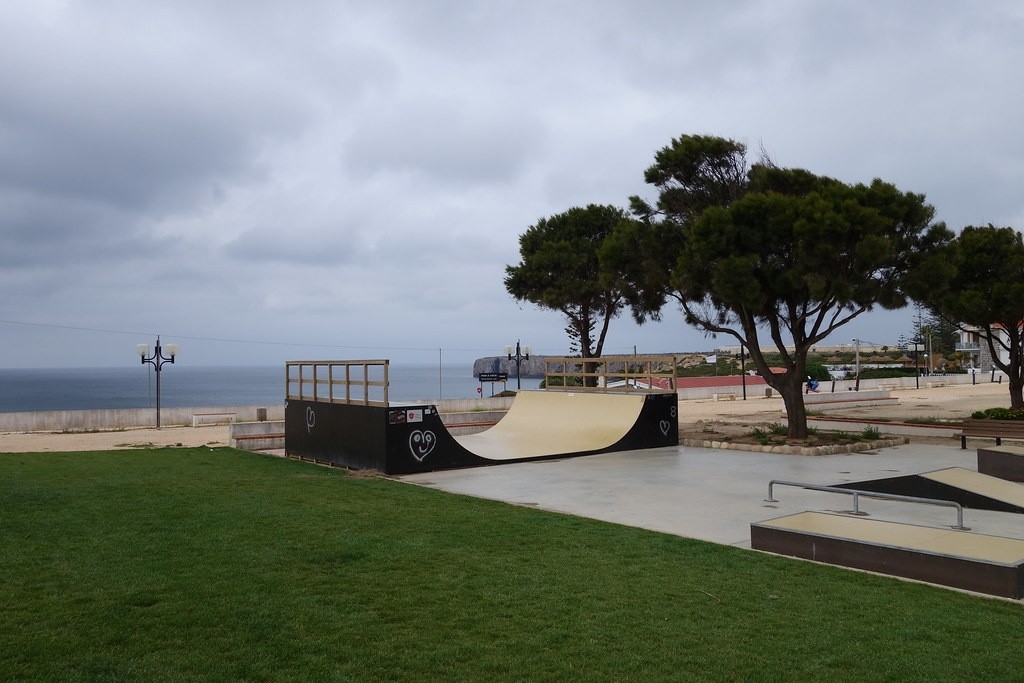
[955,418,1024,449]
[927,380,946,389]
[878,384,897,392]
[192,413,237,428]
[713,393,735,402]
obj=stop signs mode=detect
[477,387,482,393]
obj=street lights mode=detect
[908,340,924,388]
[504,338,531,389]
[135,334,178,427]
[735,344,750,400]
